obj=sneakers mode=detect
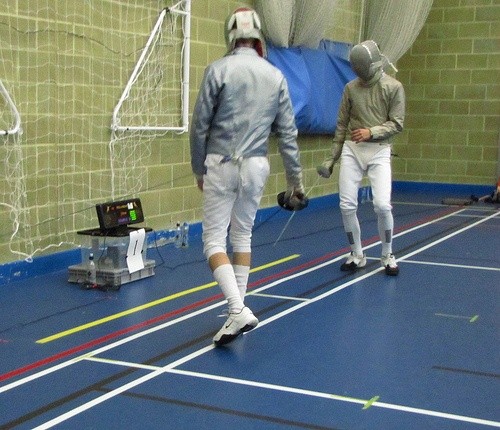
[213,306,258,346]
[340,252,366,271]
[381,253,399,276]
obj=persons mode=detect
[317,40,405,276]
[190,7,306,347]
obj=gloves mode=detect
[325,143,342,161]
[284,171,306,202]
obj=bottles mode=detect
[87,253,96,288]
[174,220,182,248]
[181,222,189,248]
